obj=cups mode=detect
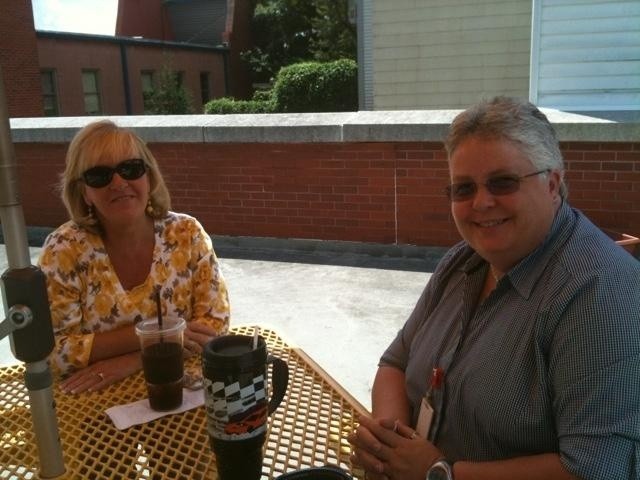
[201,333,291,453]
[134,315,187,412]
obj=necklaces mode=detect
[488,260,504,284]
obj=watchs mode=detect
[425,456,454,480]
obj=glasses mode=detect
[445,168,551,203]
[75,158,150,189]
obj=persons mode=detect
[345,91,640,480]
[36,117,232,479]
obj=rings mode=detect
[97,373,106,380]
[410,430,421,441]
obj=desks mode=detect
[0,324,374,480]
